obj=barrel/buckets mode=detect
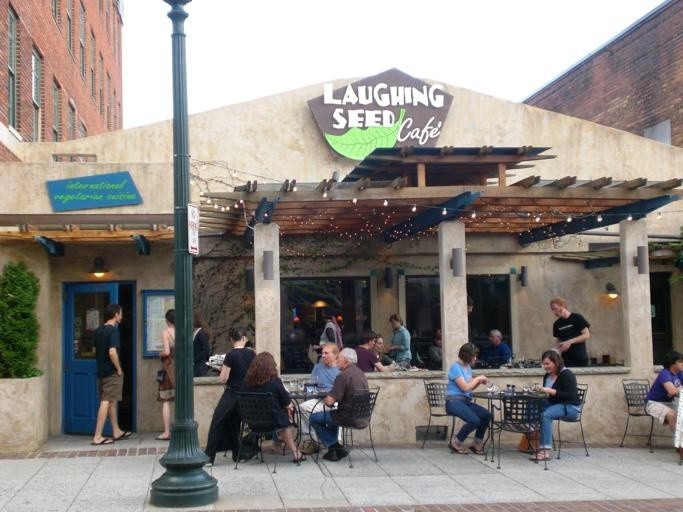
[468,446,485,454]
[92,438,114,445]
[114,431,132,440]
[449,441,468,454]
[155,434,171,440]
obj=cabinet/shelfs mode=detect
[506,384,511,393]
[511,385,516,393]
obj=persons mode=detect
[192,313,211,375]
[642,350,683,433]
[204,325,370,466]
[90,303,133,445]
[549,296,592,367]
[310,306,413,372]
[422,295,512,368]
[528,349,581,461]
[445,342,493,453]
[156,309,177,439]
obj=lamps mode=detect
[621,378,656,453]
[421,378,461,456]
[558,381,591,458]
[281,342,311,374]
[231,390,295,474]
[331,386,384,466]
[492,389,551,470]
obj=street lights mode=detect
[156,370,165,382]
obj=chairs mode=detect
[530,450,554,461]
[271,440,348,462]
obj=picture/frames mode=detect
[468,389,550,462]
[289,391,329,464]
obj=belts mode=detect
[486,383,494,395]
[290,379,299,395]
[312,376,319,396]
[298,384,305,396]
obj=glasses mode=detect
[84,257,112,278]
[384,267,393,288]
[450,248,464,277]
[605,282,621,300]
[262,251,273,281]
[632,246,649,274]
[517,266,529,286]
[246,270,253,290]
